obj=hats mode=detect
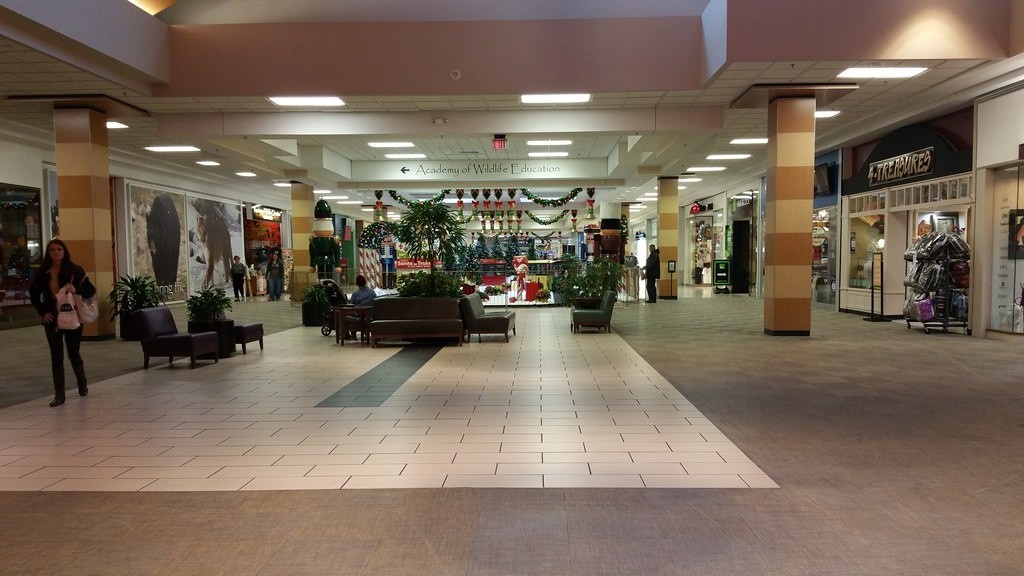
[356,275,366,286]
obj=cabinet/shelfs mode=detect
[904,245,971,334]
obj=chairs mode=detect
[211,308,264,355]
[132,307,219,368]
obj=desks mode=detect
[332,306,371,346]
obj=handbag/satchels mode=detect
[71,273,99,323]
[904,234,969,321]
[55,287,80,329]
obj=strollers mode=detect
[312,279,350,337]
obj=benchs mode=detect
[369,297,463,347]
[571,290,616,334]
[459,293,516,344]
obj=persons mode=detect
[265,252,283,301]
[230,255,246,302]
[392,267,397,286]
[644,244,660,303]
[345,275,377,340]
[29,239,96,407]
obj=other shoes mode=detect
[241,297,244,301]
[268,298,273,301]
[646,300,656,303]
[276,297,280,301]
[234,299,240,302]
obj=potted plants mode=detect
[186,283,234,359]
[301,281,331,327]
[109,274,166,341]
[551,253,629,328]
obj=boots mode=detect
[73,360,88,396]
[50,368,66,407]
